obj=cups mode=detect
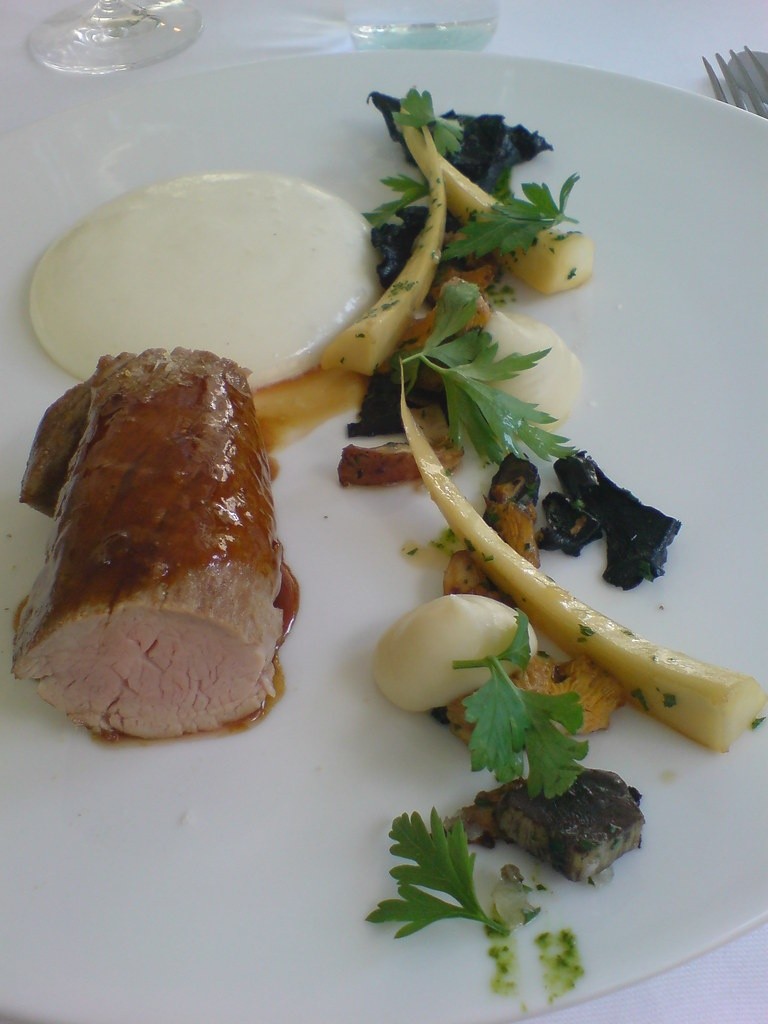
[343,1,501,52]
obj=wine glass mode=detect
[26,0,205,78]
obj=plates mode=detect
[0,52,767,1020]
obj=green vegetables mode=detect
[363,88,594,937]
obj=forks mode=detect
[701,46,768,119]
